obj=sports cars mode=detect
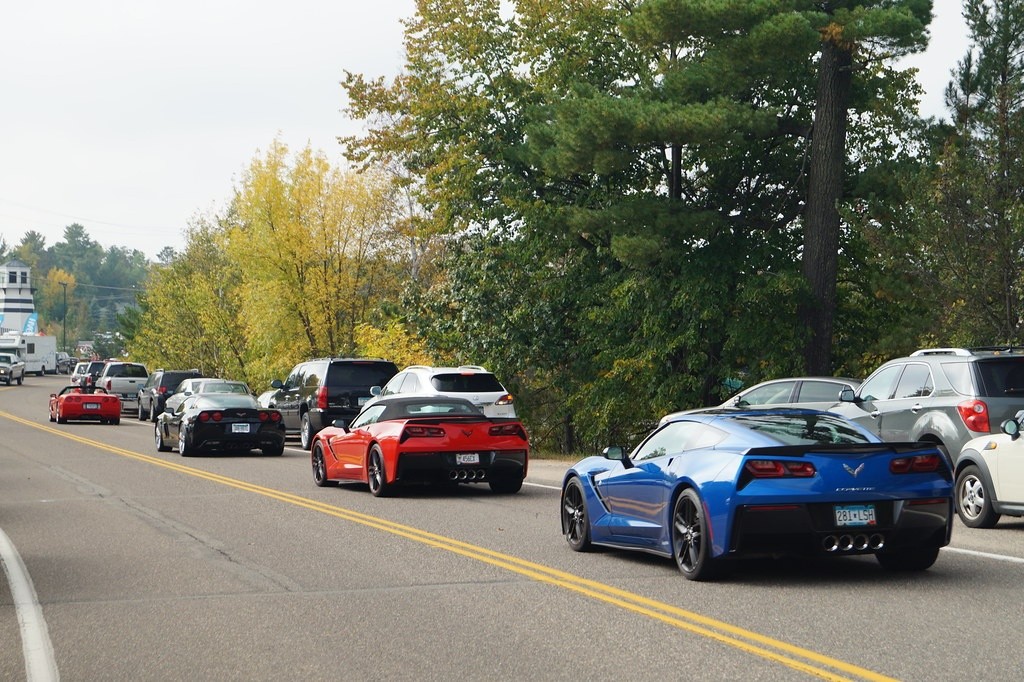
[49,386,121,425]
[561,407,955,581]
[310,397,529,497]
[155,393,286,456]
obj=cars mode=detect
[0,353,25,385]
[56,351,92,375]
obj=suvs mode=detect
[825,348,1024,528]
[137,369,204,422]
[70,361,150,414]
[268,358,400,450]
[361,365,516,419]
[658,378,860,430]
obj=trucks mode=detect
[0,331,56,376]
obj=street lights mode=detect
[59,281,67,352]
[132,285,136,313]
